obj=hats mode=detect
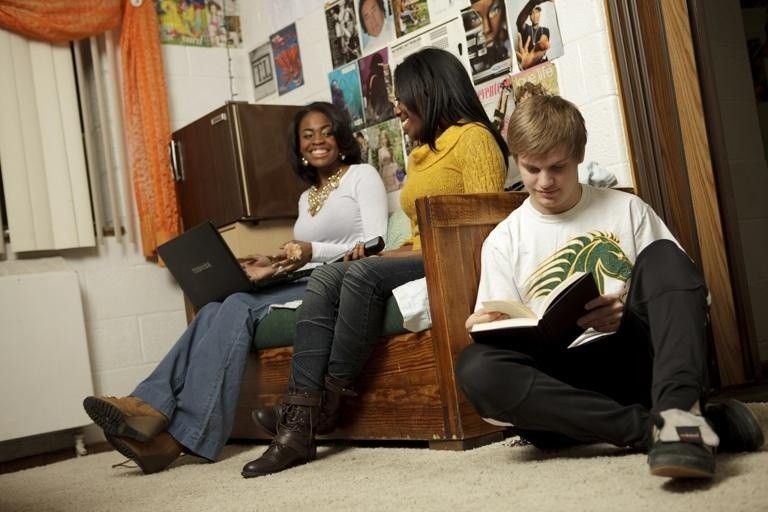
[533,6,542,11]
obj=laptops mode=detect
[156,219,314,313]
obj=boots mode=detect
[251,371,361,438]
[235,388,316,477]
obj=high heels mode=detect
[99,431,184,476]
[84,394,166,439]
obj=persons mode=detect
[396,3,418,36]
[452,93,763,483]
[515,0,550,69]
[329,78,349,113]
[358,0,396,54]
[374,129,399,191]
[81,100,390,475]
[363,53,394,119]
[239,47,509,477]
[465,0,508,47]
[354,132,370,164]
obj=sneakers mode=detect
[647,409,709,482]
[698,396,765,456]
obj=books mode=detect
[468,271,600,362]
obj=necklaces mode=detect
[308,167,344,218]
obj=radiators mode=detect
[1,255,98,459]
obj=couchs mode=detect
[178,185,638,452]
